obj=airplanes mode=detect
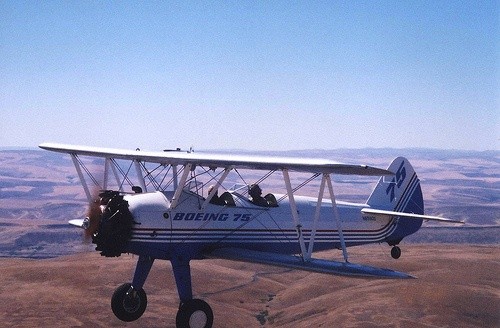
[39,142,467,328]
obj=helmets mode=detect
[248,184,262,195]
[208,185,218,196]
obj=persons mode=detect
[208,185,227,206]
[248,184,268,207]
